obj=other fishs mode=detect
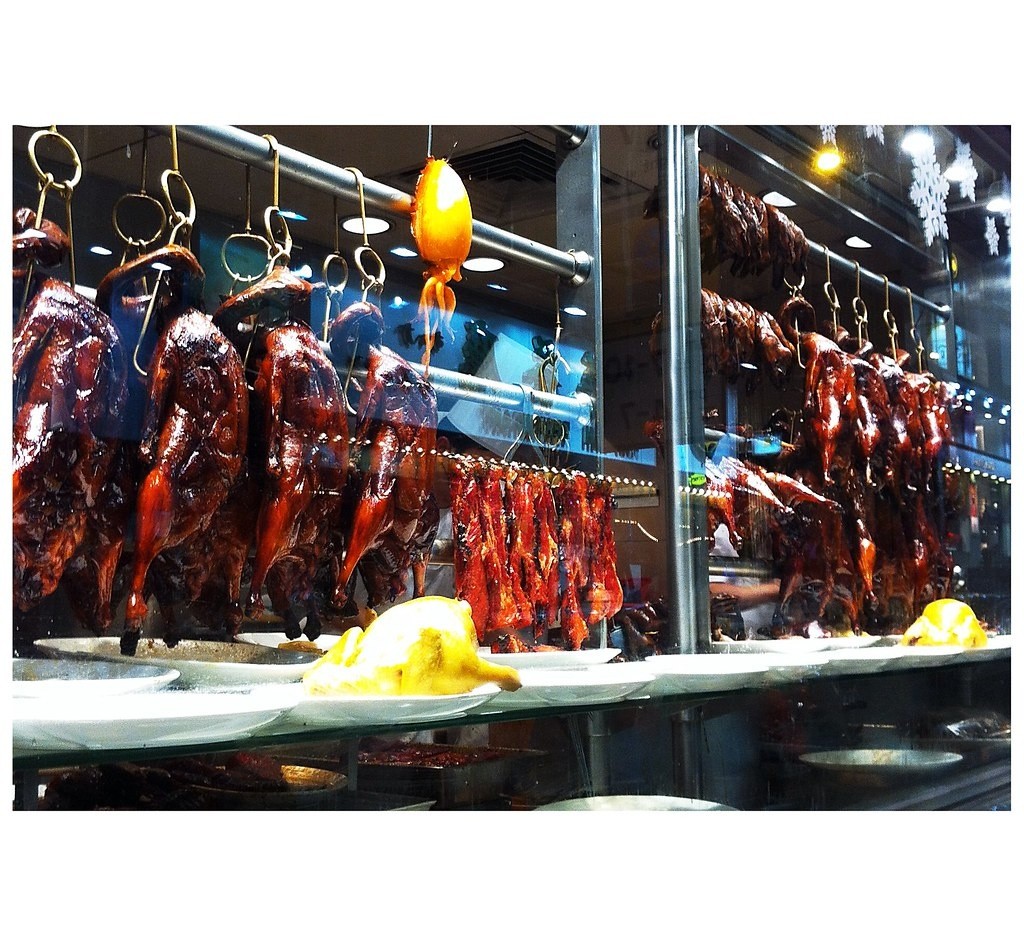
[406,157,473,380]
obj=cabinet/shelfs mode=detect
[12,124,1010,812]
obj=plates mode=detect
[33,634,318,684]
[12,696,304,749]
[711,639,780,653]
[246,679,503,722]
[830,648,901,676]
[897,645,965,668]
[800,750,962,788]
[884,636,904,642]
[518,666,655,704]
[477,645,623,670]
[232,632,342,652]
[967,635,1010,660]
[828,634,884,648]
[777,637,831,650]
[646,653,773,693]
[177,761,349,805]
[13,657,179,700]
[532,794,740,811]
[745,651,826,681]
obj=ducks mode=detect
[12,208,441,657]
[769,296,955,640]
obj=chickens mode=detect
[703,455,846,552]
[446,455,625,653]
[900,598,987,648]
[297,595,523,698]
